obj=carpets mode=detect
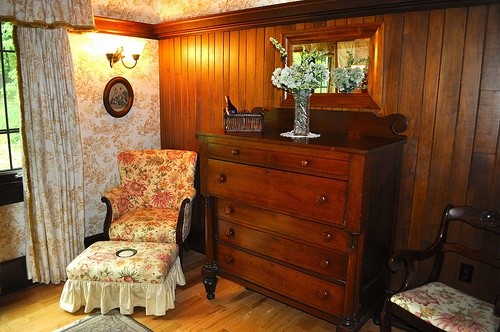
[54,309,154,332]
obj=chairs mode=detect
[379,204,500,332]
[101,149,198,267]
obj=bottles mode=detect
[224,95,237,115]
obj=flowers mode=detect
[269,36,331,90]
[331,50,370,92]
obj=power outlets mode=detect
[458,261,473,284]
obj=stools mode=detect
[60,240,186,316]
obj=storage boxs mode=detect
[223,113,264,133]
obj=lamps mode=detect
[94,37,147,70]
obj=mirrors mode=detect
[278,21,387,109]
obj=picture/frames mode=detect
[103,77,134,118]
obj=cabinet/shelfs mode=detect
[194,108,408,331]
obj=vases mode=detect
[284,87,312,136]
